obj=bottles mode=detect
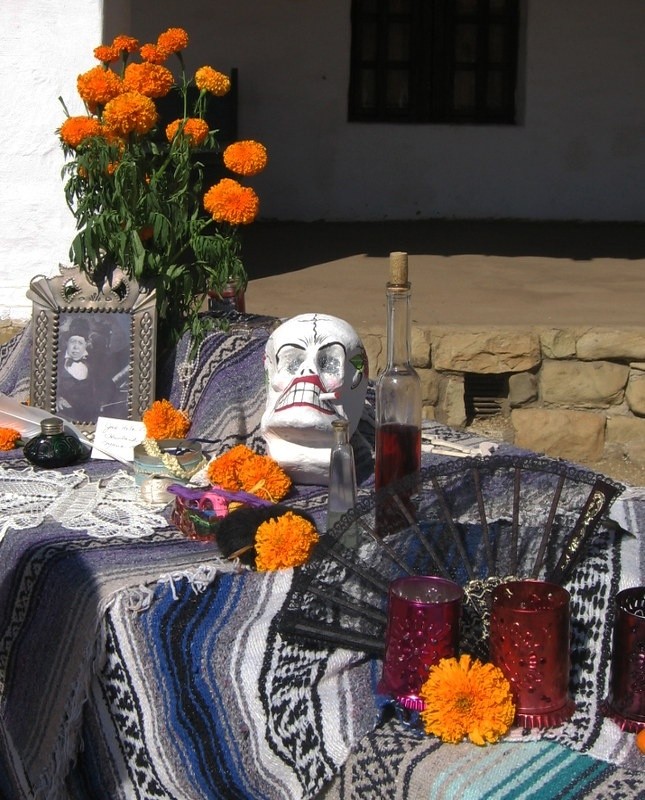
[325,418,359,552]
[24,418,82,468]
[375,282,423,537]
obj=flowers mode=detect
[54,25,268,355]
[207,443,291,505]
[416,652,516,744]
[142,399,190,441]
[255,510,319,573]
[0,426,30,451]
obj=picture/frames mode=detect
[25,264,159,441]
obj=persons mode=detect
[56,318,100,425]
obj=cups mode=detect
[208,276,245,314]
